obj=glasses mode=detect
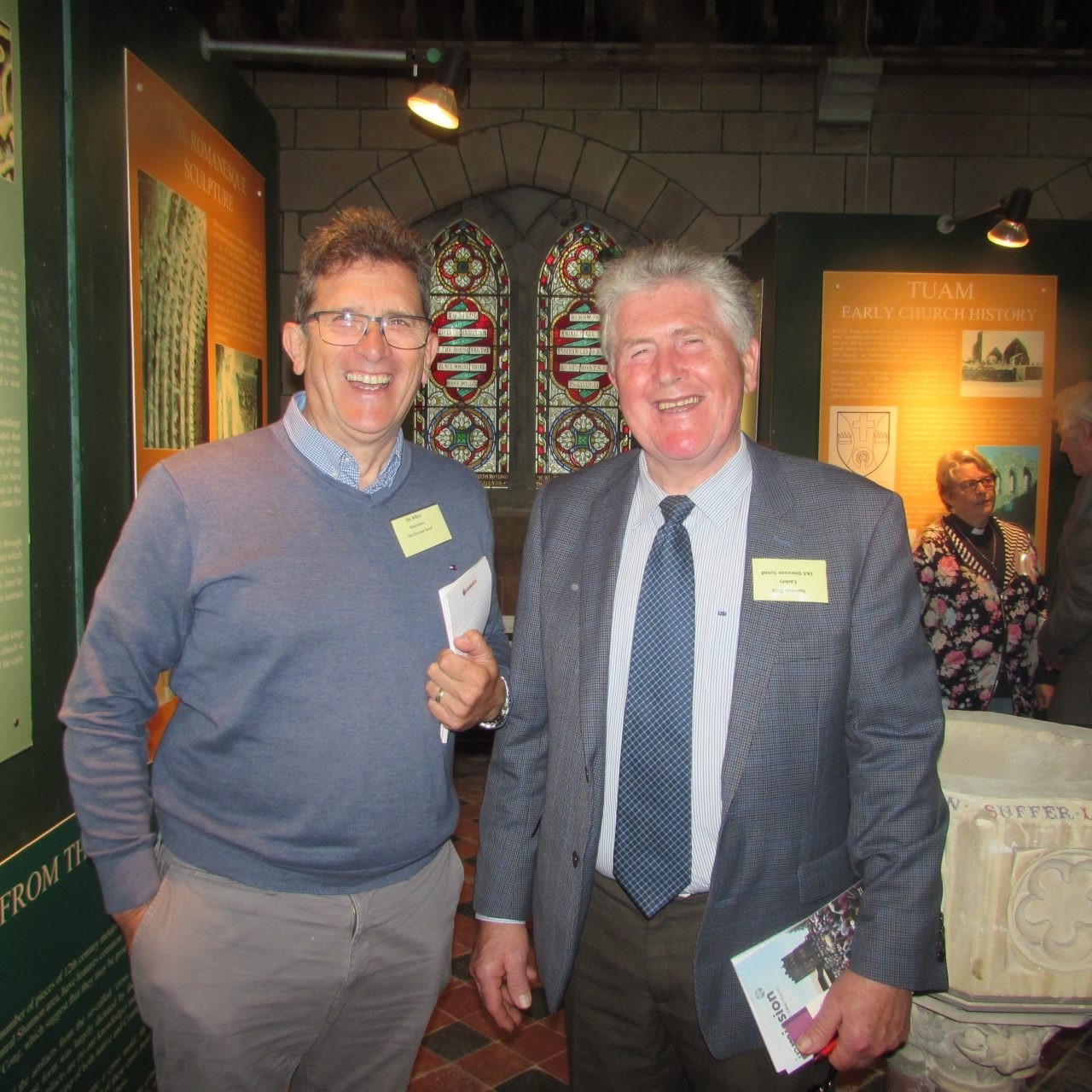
[953,474,994,492]
[303,310,434,350]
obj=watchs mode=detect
[483,679,509,727]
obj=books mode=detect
[729,878,861,1075]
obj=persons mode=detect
[60,204,512,1092]
[1035,383,1092,729]
[469,245,949,1092]
[911,448,1055,717]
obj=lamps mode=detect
[199,28,467,129]
[937,187,1032,247]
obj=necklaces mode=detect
[950,518,997,570]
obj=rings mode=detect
[434,688,445,702]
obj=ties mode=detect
[612,494,695,919]
